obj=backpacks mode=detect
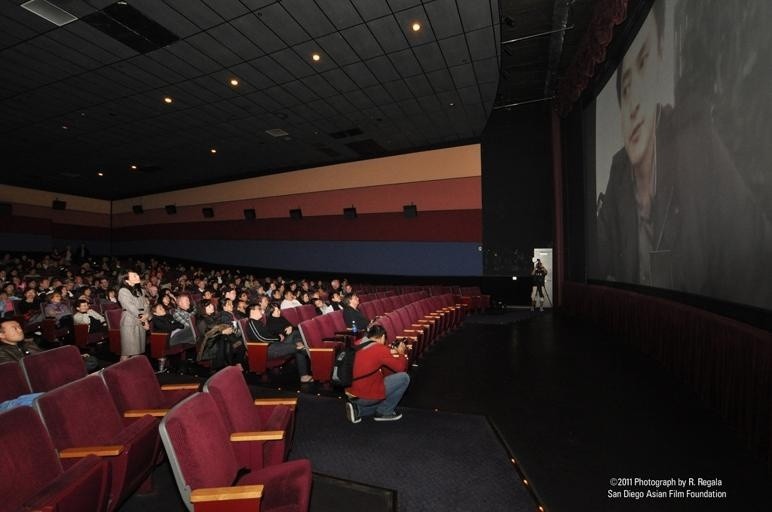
[331,341,376,387]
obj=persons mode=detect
[342,292,371,331]
[598,2,769,305]
[532,263,549,313]
[0,238,353,384]
[343,324,412,423]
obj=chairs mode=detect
[31,372,156,507]
[160,391,311,510]
[97,352,195,444]
[202,365,298,467]
[1,403,109,512]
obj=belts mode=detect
[123,308,151,313]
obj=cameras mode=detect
[392,338,408,348]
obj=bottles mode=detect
[350,320,356,341]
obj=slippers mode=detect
[300,375,317,383]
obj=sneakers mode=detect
[531,306,544,312]
[373,409,403,421]
[346,402,362,424]
